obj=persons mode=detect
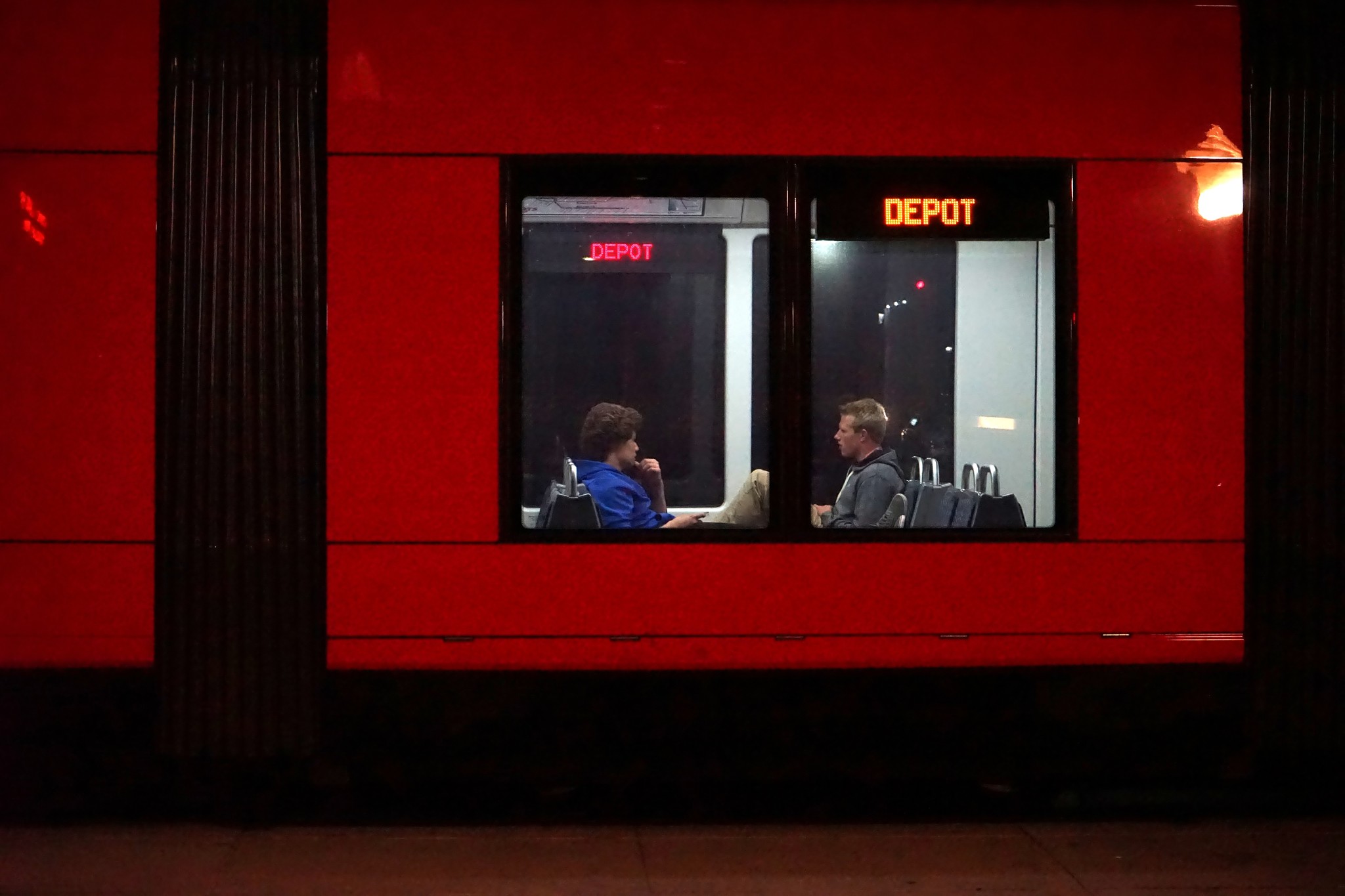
[578,403,907,527]
[813,398,907,528]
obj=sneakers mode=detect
[876,492,909,528]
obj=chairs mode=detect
[535,455,602,529]
[904,452,1027,528]
